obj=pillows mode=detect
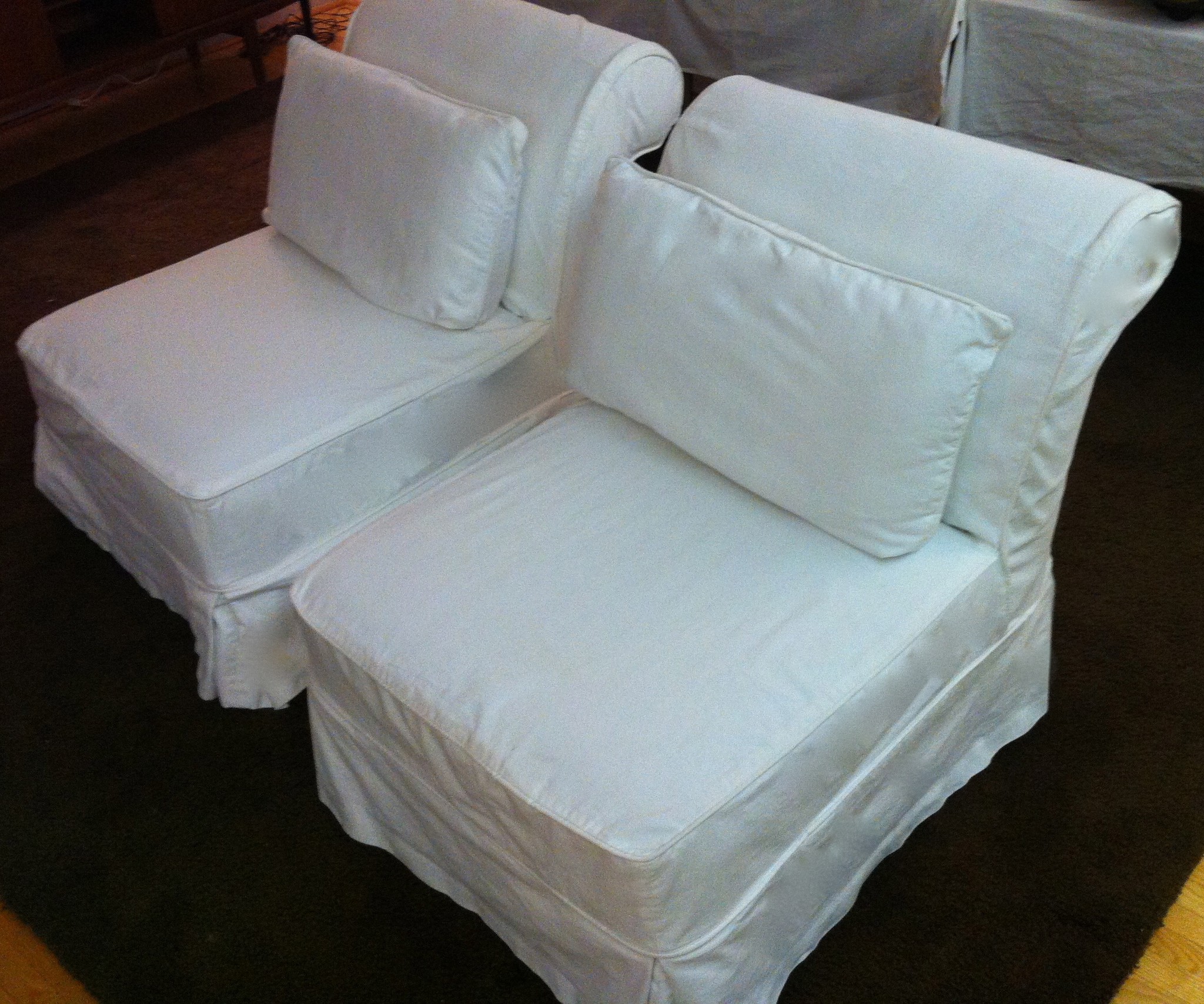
[262,34,528,329]
[570,156,1014,561]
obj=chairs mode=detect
[15,1,683,710]
[288,76,1184,1004]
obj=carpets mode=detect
[0,234,1204,1004]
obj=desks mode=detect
[535,0,1204,190]
